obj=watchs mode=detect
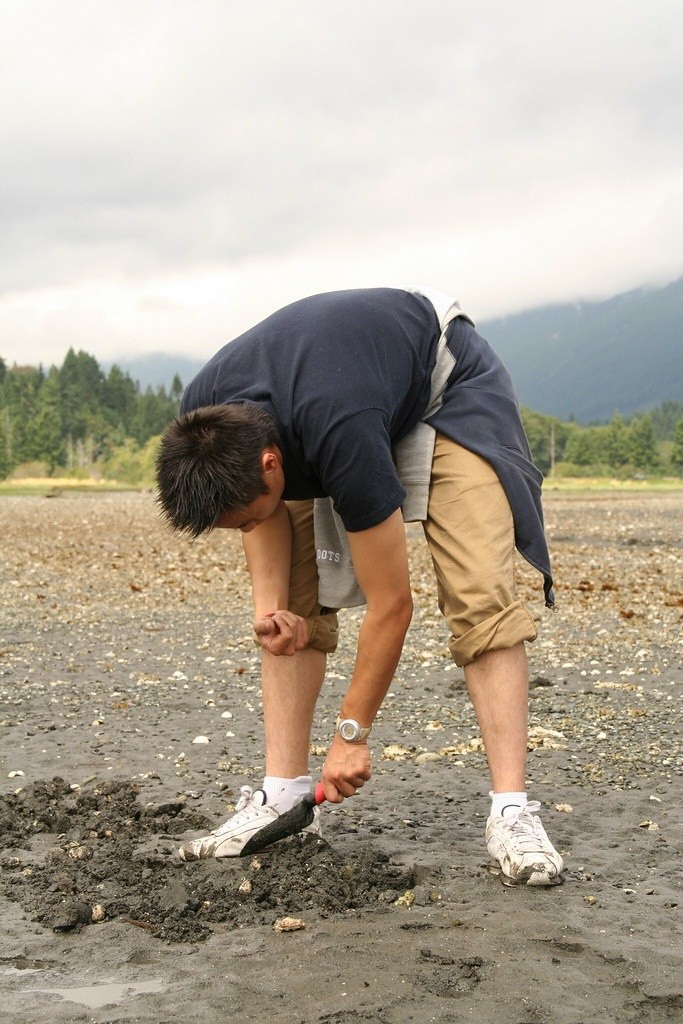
[336,715,372,743]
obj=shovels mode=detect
[239,780,335,856]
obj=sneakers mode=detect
[485,800,565,887]
[179,791,322,862]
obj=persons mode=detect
[151,285,566,887]
